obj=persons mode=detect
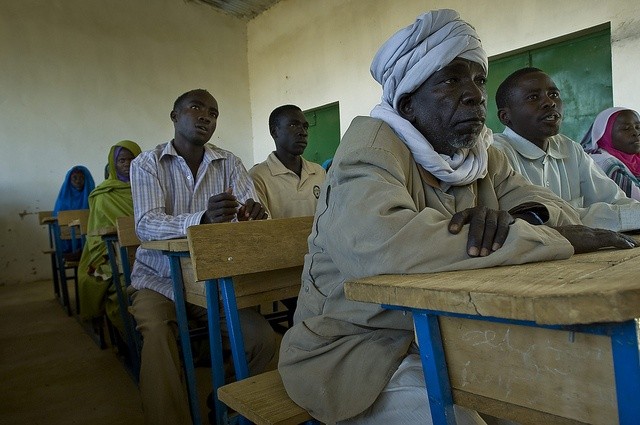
[49,165,95,262]
[248,105,329,328]
[276,9,640,425]
[491,67,640,233]
[579,107,640,201]
[125,89,276,424]
[77,139,192,347]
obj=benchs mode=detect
[38,211,58,295]
[116,217,141,248]
[58,210,84,267]
[79,212,88,236]
[187,217,313,422]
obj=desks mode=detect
[89,227,139,372]
[42,215,76,323]
[344,235,636,424]
[140,238,303,425]
